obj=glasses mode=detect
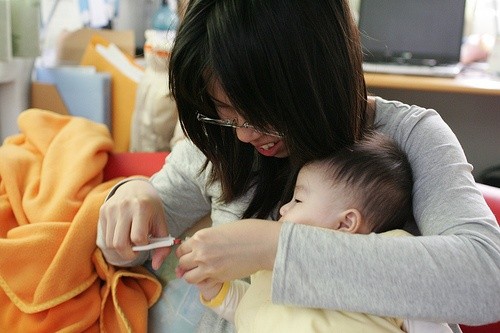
[195,110,289,137]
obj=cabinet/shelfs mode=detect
[0,0,40,144]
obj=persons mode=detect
[175,133,454,333]
[96,0,500,333]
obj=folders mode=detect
[33,36,146,153]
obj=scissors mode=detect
[132,235,185,252]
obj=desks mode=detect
[360,56,500,103]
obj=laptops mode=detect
[358,0,466,78]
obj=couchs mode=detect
[0,145,500,333]
